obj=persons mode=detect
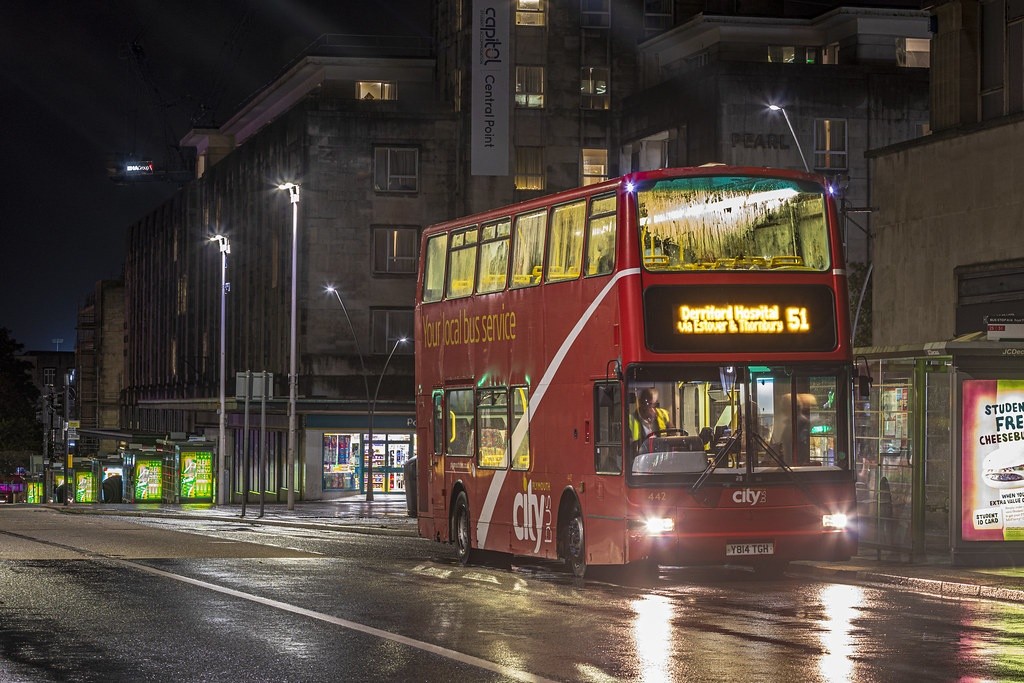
[626,387,678,454]
[761,393,810,468]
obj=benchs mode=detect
[435,417,524,457]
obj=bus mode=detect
[412,163,875,577]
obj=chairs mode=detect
[424,255,804,301]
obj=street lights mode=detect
[278,181,301,511]
[211,233,229,504]
[325,284,409,501]
